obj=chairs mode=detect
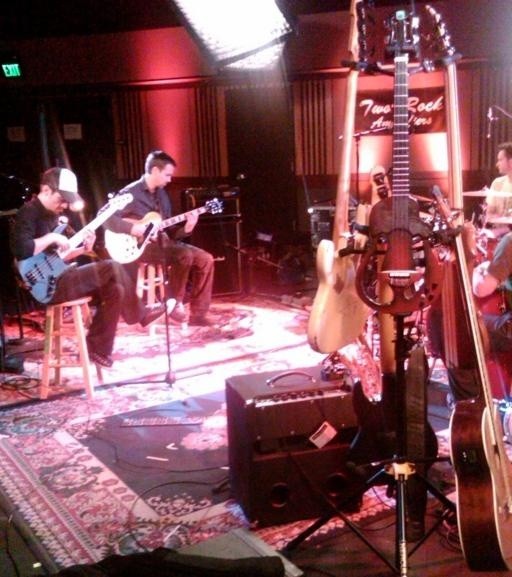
[188,312,224,326]
[163,297,189,322]
[140,298,177,331]
[86,337,114,368]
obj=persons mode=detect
[446,231,512,410]
[484,143,512,229]
[96,150,221,326]
[8,166,177,369]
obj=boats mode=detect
[186,220,243,298]
[225,367,361,520]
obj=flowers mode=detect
[482,111,498,142]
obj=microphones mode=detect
[39,295,103,401]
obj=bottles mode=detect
[42,167,84,204]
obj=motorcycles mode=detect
[276,316,456,573]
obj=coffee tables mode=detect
[488,216,512,226]
[462,189,511,198]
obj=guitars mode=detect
[18,194,135,304]
[425,2,483,364]
[356,2,441,317]
[310,2,373,355]
[434,188,512,570]
[311,167,438,477]
[105,197,224,264]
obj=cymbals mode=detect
[462,189,511,198]
[488,216,512,226]
